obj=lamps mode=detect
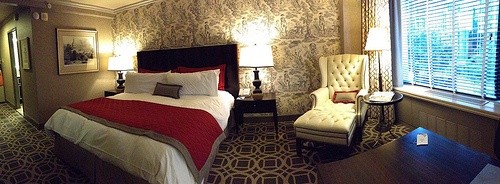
[365,28,390,92]
[239,44,274,99]
[108,55,135,90]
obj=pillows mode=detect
[125,64,226,97]
[333,90,359,103]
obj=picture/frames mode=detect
[56,28,100,75]
[19,37,32,70]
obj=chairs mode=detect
[294,54,370,155]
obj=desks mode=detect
[363,92,404,147]
[316,126,500,184]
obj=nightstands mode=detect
[235,95,278,133]
[104,90,118,97]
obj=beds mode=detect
[45,44,238,184]
